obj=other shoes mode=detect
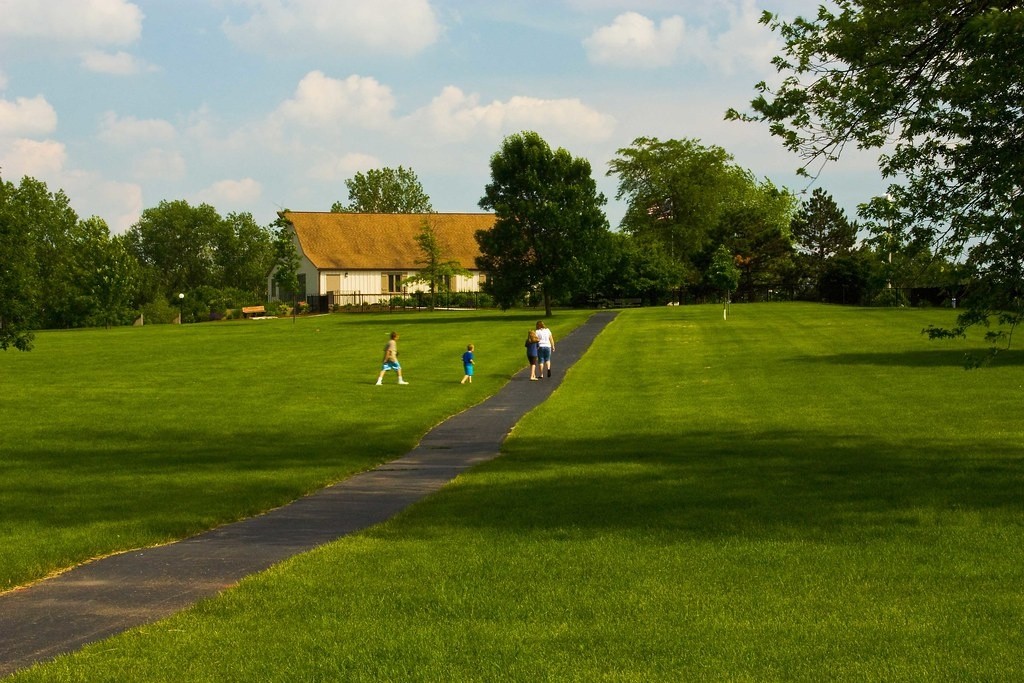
[375,382,383,386]
[397,381,410,385]
[538,375,543,378]
[534,375,537,378]
[530,378,539,381]
[547,369,551,377]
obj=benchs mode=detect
[242,306,267,318]
[615,299,641,308]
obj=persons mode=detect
[525,330,540,381]
[460,344,475,384]
[375,332,409,385]
[535,320,555,378]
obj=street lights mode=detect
[179,293,184,324]
